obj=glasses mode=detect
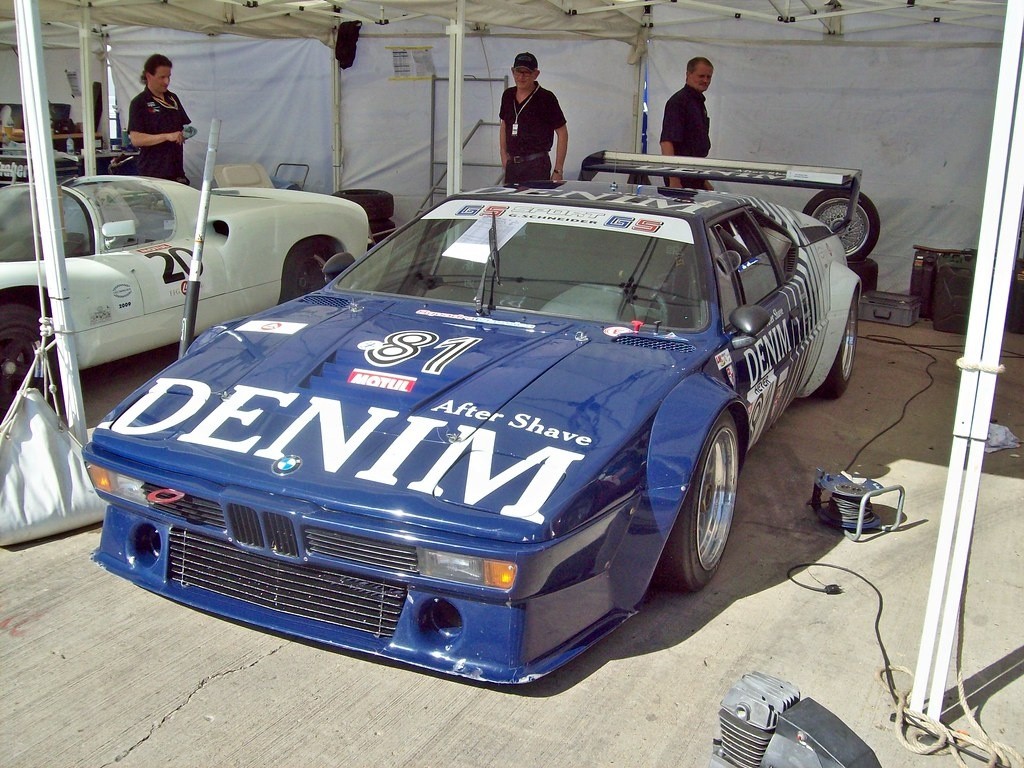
[515,70,532,77]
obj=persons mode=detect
[127,54,192,187]
[498,51,568,184]
[659,57,715,191]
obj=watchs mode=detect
[554,169,563,175]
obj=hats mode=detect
[514,53,538,74]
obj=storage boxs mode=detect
[857,291,920,326]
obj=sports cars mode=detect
[0,171,371,421]
[77,146,867,692]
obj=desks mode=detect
[9,132,104,151]
